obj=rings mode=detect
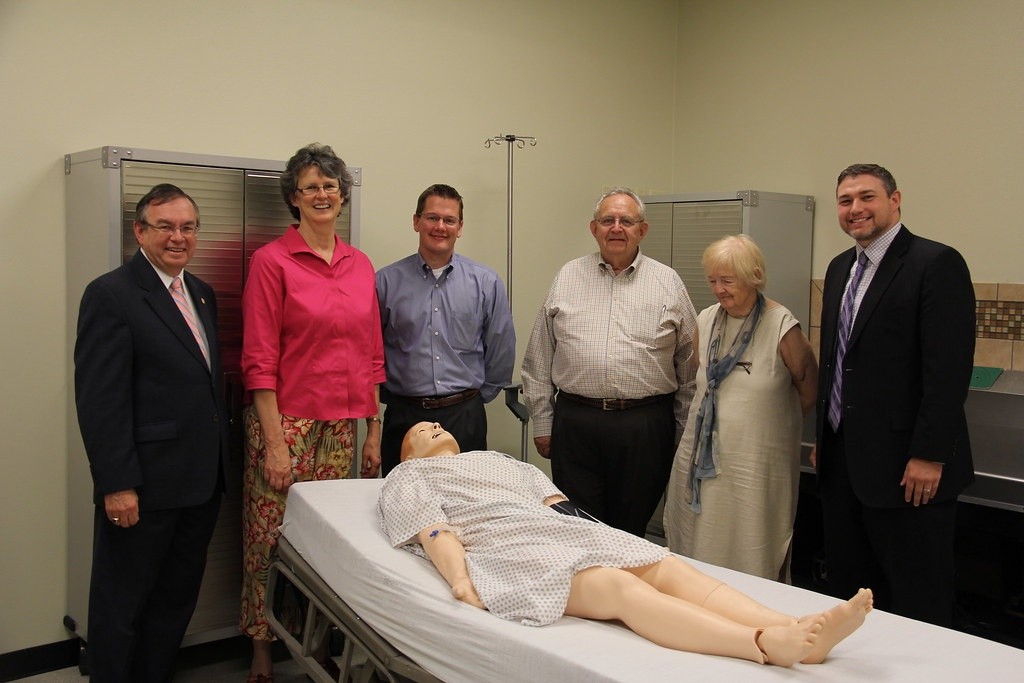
[924,489,930,492]
[112,518,119,523]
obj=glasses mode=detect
[146,222,199,236]
[597,217,642,227]
[419,213,460,226]
[296,183,340,194]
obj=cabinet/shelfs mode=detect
[63,144,362,653]
[630,189,812,339]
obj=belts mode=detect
[560,390,672,411]
[403,389,480,409]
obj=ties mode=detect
[828,251,868,434]
[170,278,209,370]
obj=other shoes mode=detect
[320,658,353,683]
[247,672,274,683]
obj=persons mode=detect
[809,163,977,626]
[521,187,698,539]
[375,184,516,478]
[241,145,386,683]
[73,183,234,683]
[663,233,819,583]
[378,420,873,667]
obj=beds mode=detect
[254,473,1023,683]
